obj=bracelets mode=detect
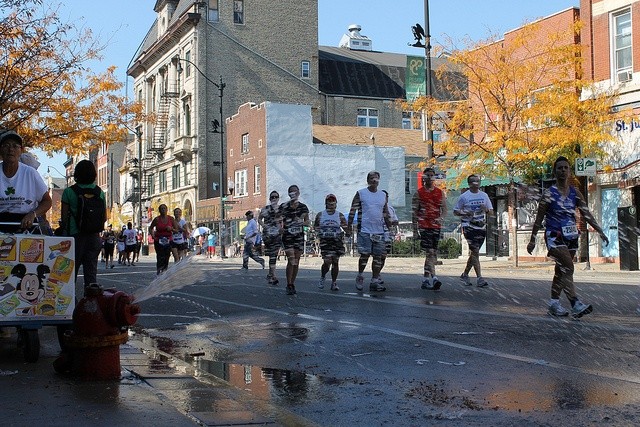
[30,209,37,217]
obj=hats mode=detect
[107,224,112,228]
[122,225,126,229]
[0,131,22,146]
[326,194,336,200]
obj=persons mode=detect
[412,167,448,291]
[313,194,348,291]
[208,229,216,259]
[189,234,207,258]
[240,210,265,271]
[54,219,62,236]
[454,174,494,287]
[526,156,609,318]
[345,171,392,292]
[170,207,188,264]
[99,221,143,269]
[148,203,179,276]
[274,185,310,295]
[377,190,398,284]
[59,160,107,306]
[0,130,53,236]
[258,190,283,285]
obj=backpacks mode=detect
[70,185,105,233]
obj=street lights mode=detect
[47,166,75,188]
[109,116,142,229]
[176,58,226,257]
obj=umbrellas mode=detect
[190,227,210,237]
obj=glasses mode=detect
[270,195,278,199]
[1,144,22,149]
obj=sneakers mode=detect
[262,259,265,269]
[356,276,364,290]
[118,260,122,264]
[123,262,127,266]
[266,276,279,285]
[378,275,383,284]
[239,267,248,271]
[330,283,339,291]
[370,282,386,292]
[286,285,296,295]
[421,281,433,290]
[319,278,326,289]
[476,277,488,287]
[433,279,441,290]
[128,262,130,267]
[571,301,592,318]
[131,263,136,266]
[547,303,568,317]
[110,265,114,269]
[459,274,472,286]
[106,268,109,269]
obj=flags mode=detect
[404,53,427,102]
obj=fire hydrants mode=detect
[72,283,139,382]
